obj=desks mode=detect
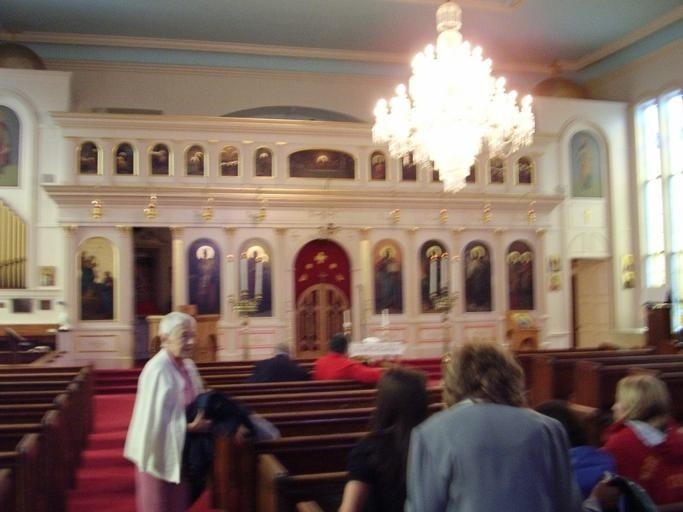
[146,315,221,361]
[347,343,410,364]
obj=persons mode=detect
[100,271,112,316]
[121,312,217,512]
[603,373,682,512]
[315,332,385,386]
[82,253,98,291]
[401,338,621,512]
[537,393,617,503]
[248,340,308,382]
[336,360,432,512]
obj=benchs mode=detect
[512,344,682,421]
[191,358,444,512]
[0,359,97,512]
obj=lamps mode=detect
[371,0,535,193]
[90,200,538,226]
